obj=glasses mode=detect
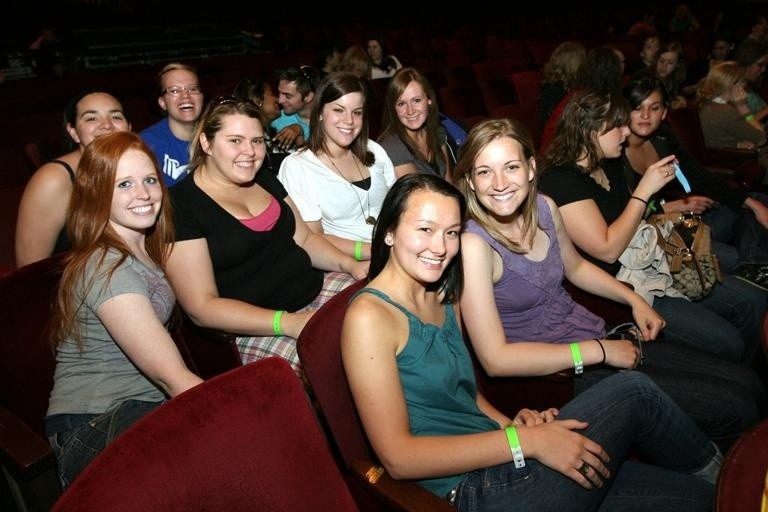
[599,322,646,371]
[160,83,202,98]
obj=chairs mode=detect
[400,22,653,164]
[715,421,765,510]
[1,248,570,512]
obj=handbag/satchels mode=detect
[648,211,724,302]
